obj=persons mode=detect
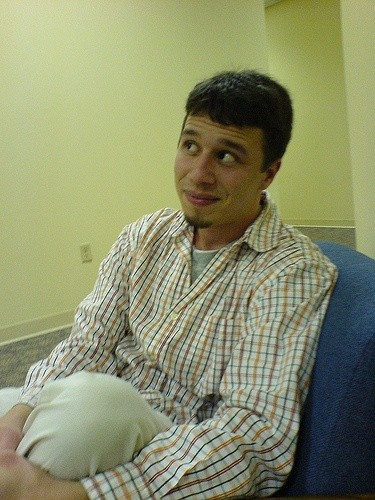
[0,68,339,500]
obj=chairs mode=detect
[269,240,375,500]
